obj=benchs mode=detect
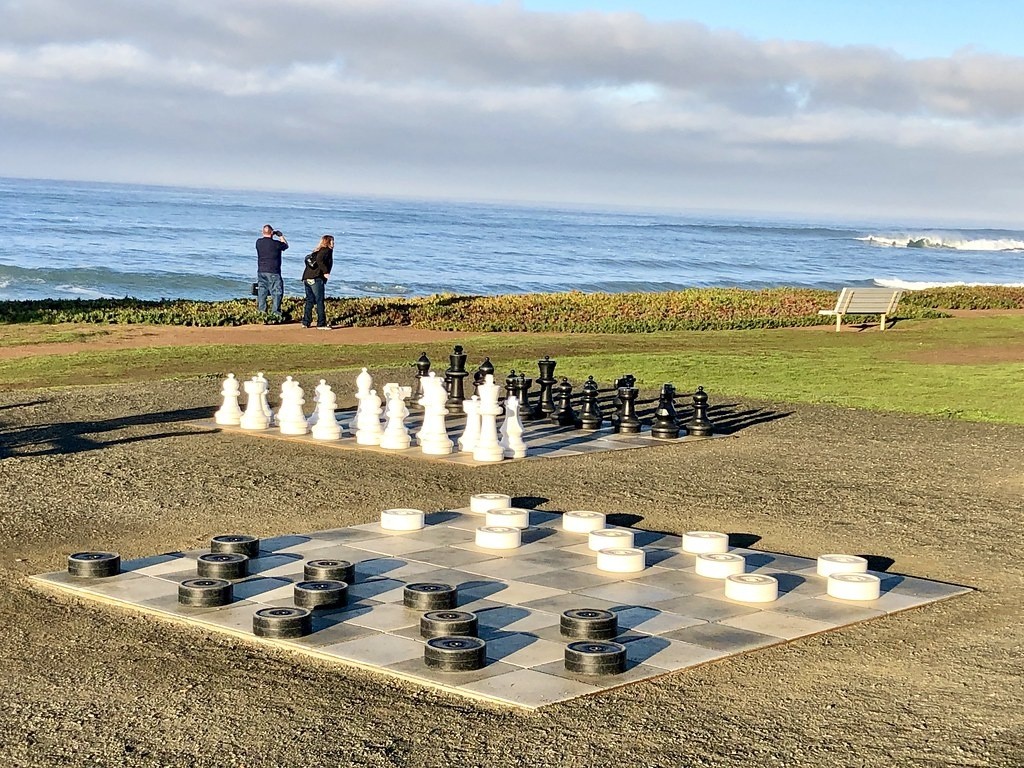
[818,287,903,333]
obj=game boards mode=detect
[182,396,736,467]
[26,501,975,713]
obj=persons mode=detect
[256,225,288,315]
[301,235,334,329]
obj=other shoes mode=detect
[301,322,307,328]
[317,326,332,330]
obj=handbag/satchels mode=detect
[305,255,318,270]
